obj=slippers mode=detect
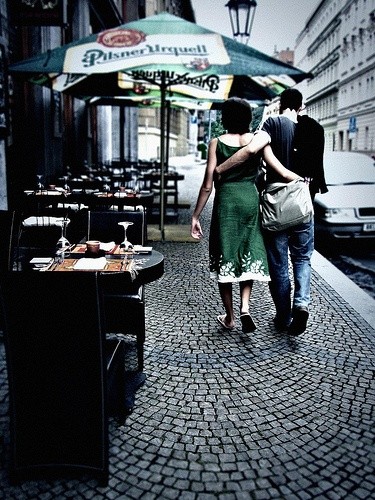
[240,312,257,333]
[217,314,235,329]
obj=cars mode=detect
[313,150,375,251]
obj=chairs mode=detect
[0,157,175,487]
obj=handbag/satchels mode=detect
[261,174,314,231]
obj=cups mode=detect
[111,181,145,201]
[49,185,56,189]
[85,240,101,253]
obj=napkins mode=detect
[99,241,116,252]
[29,257,52,264]
[72,257,106,271]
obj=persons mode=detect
[190,97,314,334]
[213,88,329,339]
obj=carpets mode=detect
[143,223,200,242]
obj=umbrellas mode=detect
[1,11,316,243]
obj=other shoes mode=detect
[273,315,288,332]
[288,308,308,336]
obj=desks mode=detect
[67,177,115,189]
[30,189,99,211]
[141,173,185,213]
[0,246,166,388]
[93,188,156,214]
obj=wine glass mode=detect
[55,218,71,258]
[118,221,134,260]
[36,175,44,192]
